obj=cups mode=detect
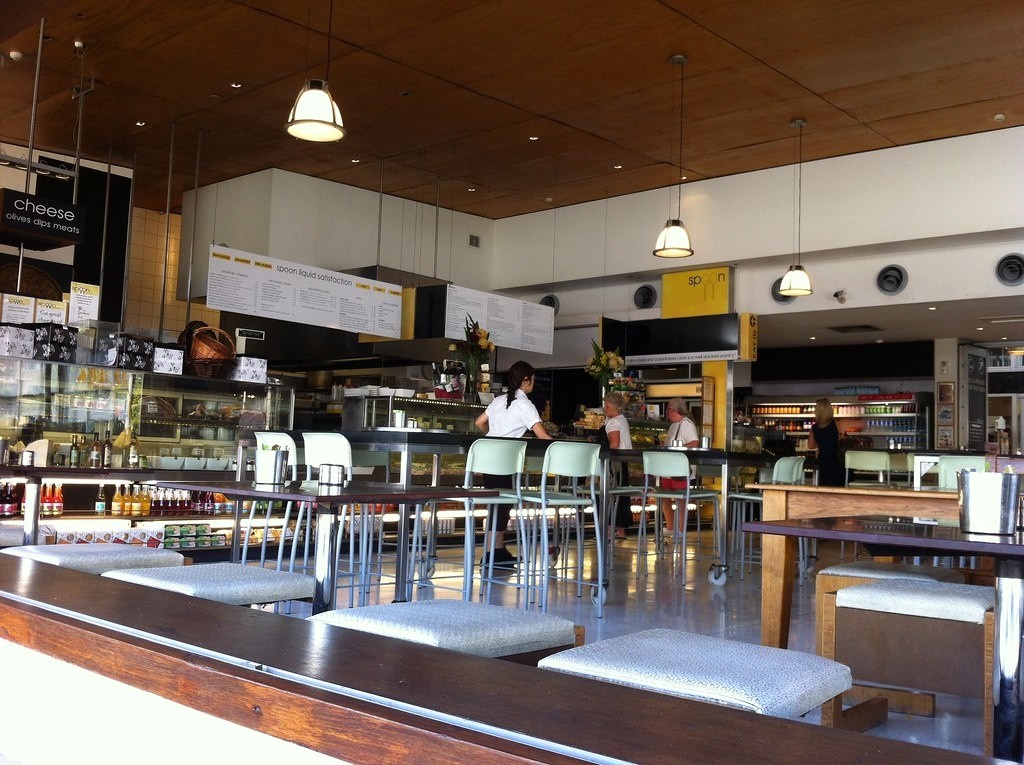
[21,450,34,466]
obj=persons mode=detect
[475,361,553,570]
[662,397,699,539]
[587,391,633,542]
[808,398,843,487]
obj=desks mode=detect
[913,452,1024,570]
[744,483,959,648]
[248,441,726,603]
[740,515,1023,765]
[155,480,500,617]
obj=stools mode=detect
[100,562,317,608]
[815,560,997,758]
[0,544,193,574]
[304,598,586,662]
[536,628,852,720]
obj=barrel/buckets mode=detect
[956,469,1020,536]
[252,447,289,485]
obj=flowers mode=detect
[608,376,647,390]
[584,337,626,384]
[448,312,496,361]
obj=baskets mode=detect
[178,320,237,379]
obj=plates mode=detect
[343,385,388,396]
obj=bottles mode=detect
[111,484,251,517]
[20,484,64,517]
[128,435,140,468]
[0,483,18,517]
[69,430,113,469]
[95,485,107,516]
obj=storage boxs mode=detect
[0,281,185,384]
[344,384,416,398]
[226,354,268,383]
[37,522,227,549]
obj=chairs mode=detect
[939,455,1010,493]
[727,455,806,586]
[408,440,719,618]
[907,453,914,487]
[985,443,1024,474]
[844,451,890,487]
[242,432,390,616]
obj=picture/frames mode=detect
[937,382,955,448]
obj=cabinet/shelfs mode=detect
[745,399,930,450]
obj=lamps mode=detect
[653,55,694,259]
[778,119,813,297]
[282,1,346,143]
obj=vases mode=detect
[599,374,610,408]
[466,358,479,394]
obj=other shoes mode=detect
[660,526,673,537]
[671,530,683,539]
[481,547,522,568]
[593,534,627,542]
